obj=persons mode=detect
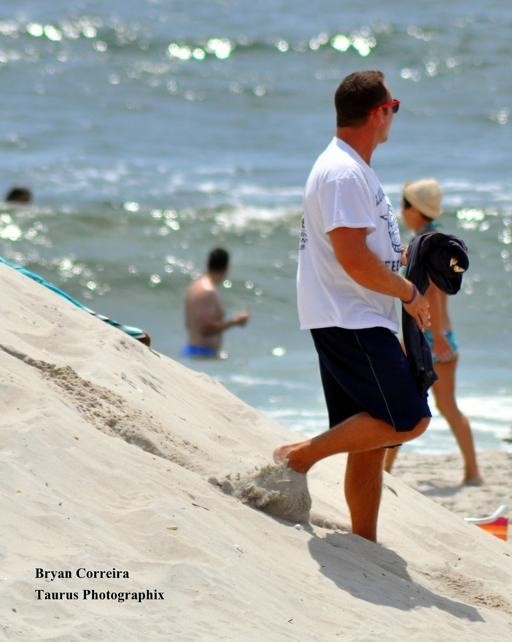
[180,248,249,358]
[273,71,433,544]
[384,177,481,488]
[6,187,32,201]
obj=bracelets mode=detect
[402,283,418,304]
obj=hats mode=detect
[403,180,442,220]
[423,241,468,294]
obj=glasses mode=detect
[403,197,411,209]
[373,99,400,128]
